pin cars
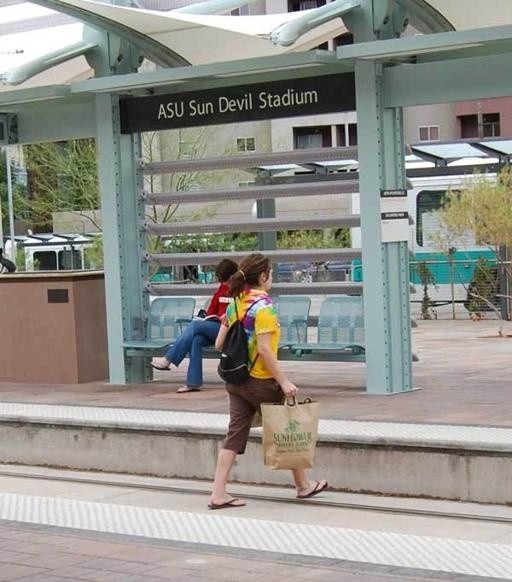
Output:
[277,261,349,281]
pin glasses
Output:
[218,277,227,281]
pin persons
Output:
[149,257,241,394]
[204,252,330,509]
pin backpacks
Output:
[218,296,263,387]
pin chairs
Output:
[120,296,366,363]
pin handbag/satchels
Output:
[260,395,321,470]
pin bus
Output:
[1,231,220,284]
[349,173,500,312]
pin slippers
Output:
[208,496,247,510]
[150,361,171,371]
[297,478,328,499]
[176,386,200,393]
